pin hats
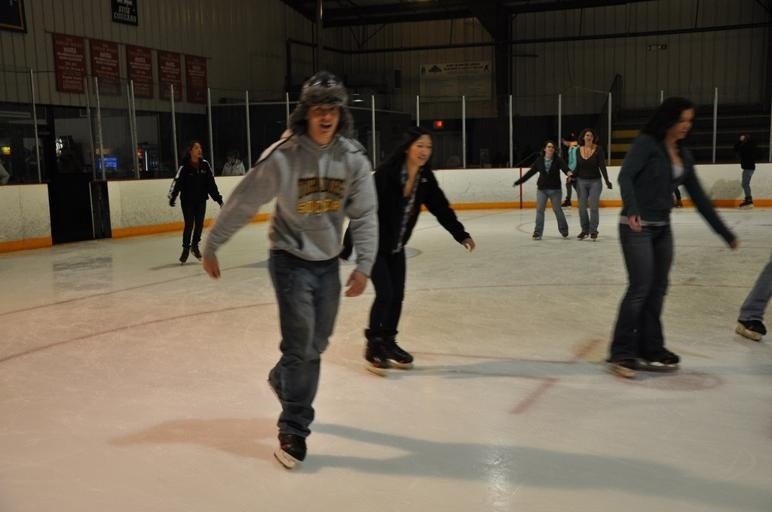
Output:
[289,72,354,139]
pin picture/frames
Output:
[0,0,27,33]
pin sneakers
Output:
[561,200,571,207]
[387,336,413,363]
[278,432,306,461]
[640,347,679,365]
[578,232,588,238]
[609,355,640,371]
[365,335,392,369]
[191,243,202,258]
[737,320,767,335]
[591,234,597,238]
[180,249,189,262]
[740,196,752,207]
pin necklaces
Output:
[582,144,593,156]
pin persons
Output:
[672,188,684,206]
[221,146,246,175]
[571,127,612,238]
[735,132,755,208]
[603,96,741,371]
[26,144,44,170]
[735,258,770,335]
[202,69,382,461]
[338,127,475,370]
[511,140,574,237]
[166,139,225,263]
[560,135,580,207]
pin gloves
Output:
[568,175,574,184]
[607,183,612,189]
